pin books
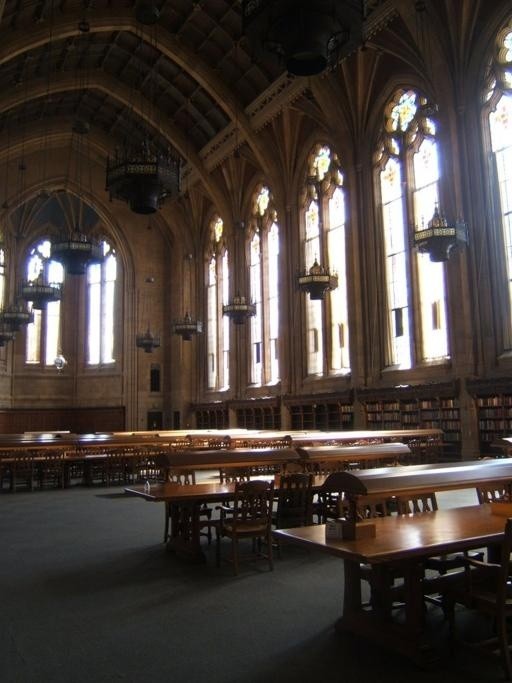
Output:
[342,396,511,442]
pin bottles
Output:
[144,481,151,490]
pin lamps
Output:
[139,319,165,355]
[106,1,182,216]
[218,286,262,326]
[291,253,344,306]
[0,41,60,345]
[169,306,205,346]
[406,196,469,269]
[49,1,109,277]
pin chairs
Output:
[135,491,338,579]
[0,433,512,491]
[339,491,511,683]
